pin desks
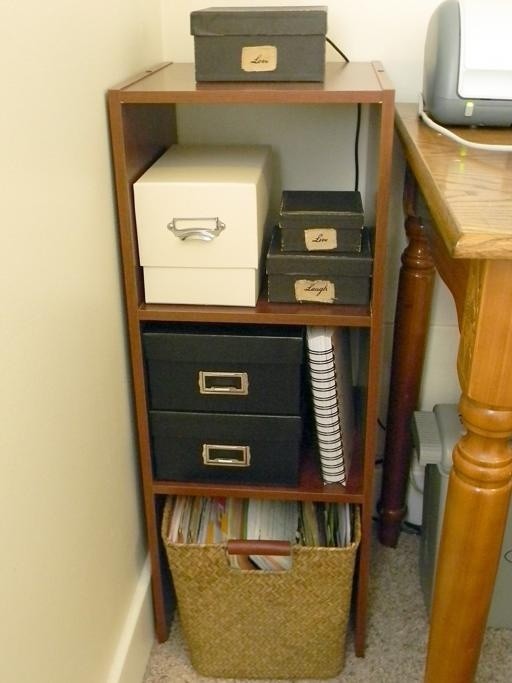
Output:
[376,98,512,683]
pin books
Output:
[308,326,355,487]
[166,494,352,573]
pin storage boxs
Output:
[192,6,327,82]
[147,411,305,491]
[281,190,365,253]
[133,142,271,309]
[264,225,373,308]
[141,330,306,417]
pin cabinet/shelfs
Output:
[105,60,395,504]
